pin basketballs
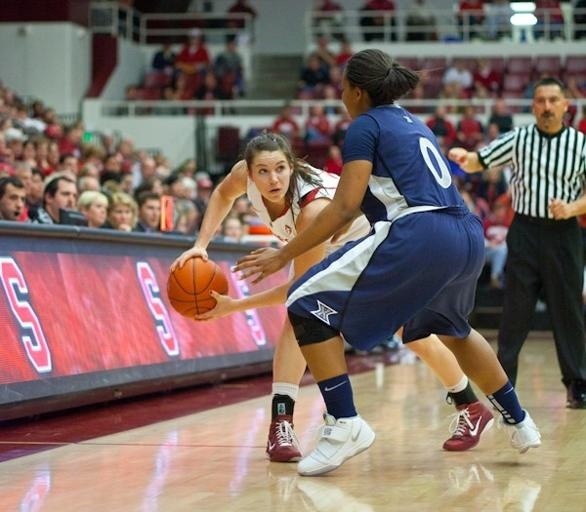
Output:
[167,257,229,318]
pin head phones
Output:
[0,179,20,217]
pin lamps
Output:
[511,14,537,27]
[511,2,536,12]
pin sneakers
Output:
[266,415,302,461]
[298,412,376,476]
[566,384,586,409]
[498,409,541,453]
[443,401,494,451]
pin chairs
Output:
[126,50,586,99]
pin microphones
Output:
[28,208,44,223]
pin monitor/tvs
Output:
[59,208,88,226]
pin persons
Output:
[0,0,586,292]
[447,74,586,409]
[230,47,543,477]
[170,132,495,463]
